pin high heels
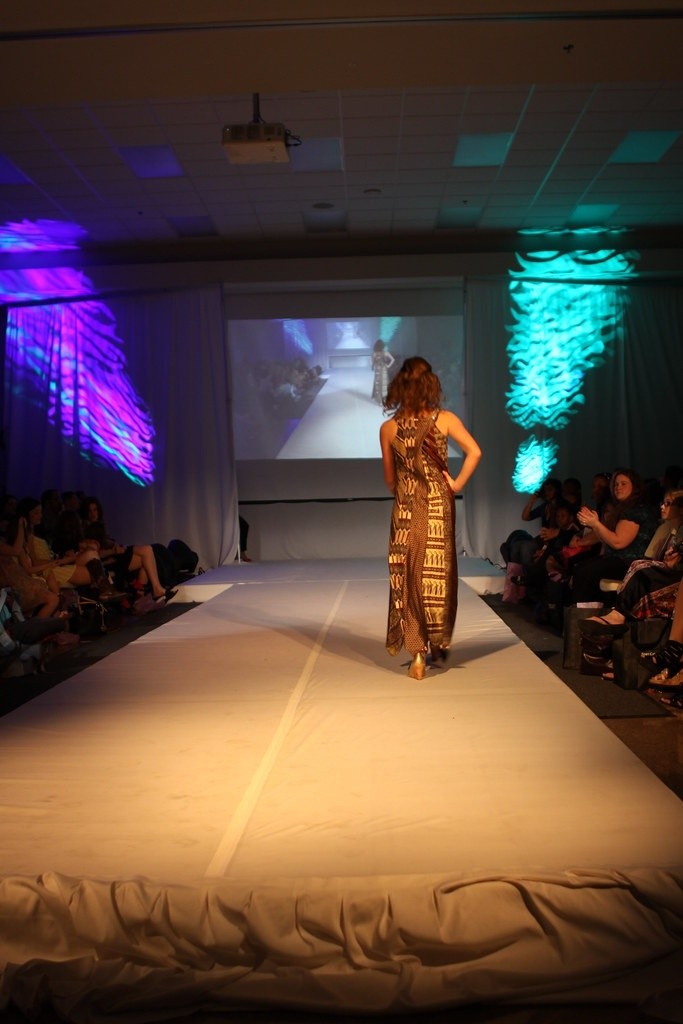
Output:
[408,652,426,680]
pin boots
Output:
[85,558,128,601]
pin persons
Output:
[239,515,251,562]
[0,489,179,655]
[370,339,396,406]
[500,467,683,696]
[380,356,482,681]
[239,357,322,401]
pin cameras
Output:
[602,472,613,480]
[535,491,546,498]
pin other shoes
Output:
[241,557,250,563]
[153,589,178,605]
[502,562,682,717]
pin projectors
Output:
[221,123,291,164]
